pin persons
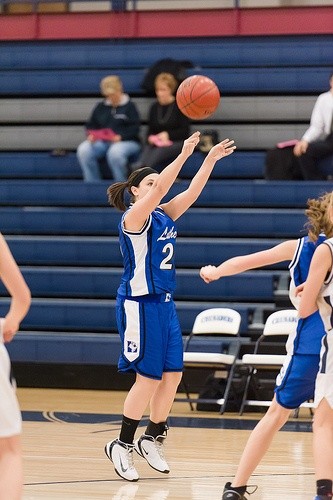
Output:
[76,75,143,184]
[0,230,32,500]
[294,71,333,181]
[294,192,333,500]
[131,72,191,174]
[200,230,327,498]
[105,130,237,481]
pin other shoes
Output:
[221,482,258,500]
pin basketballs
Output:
[175,74,221,121]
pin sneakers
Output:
[133,433,170,474]
[104,437,140,482]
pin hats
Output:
[100,76,121,95]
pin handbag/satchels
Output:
[264,150,293,179]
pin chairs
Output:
[239,309,313,419]
[182,308,243,415]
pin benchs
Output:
[0,35,333,392]
